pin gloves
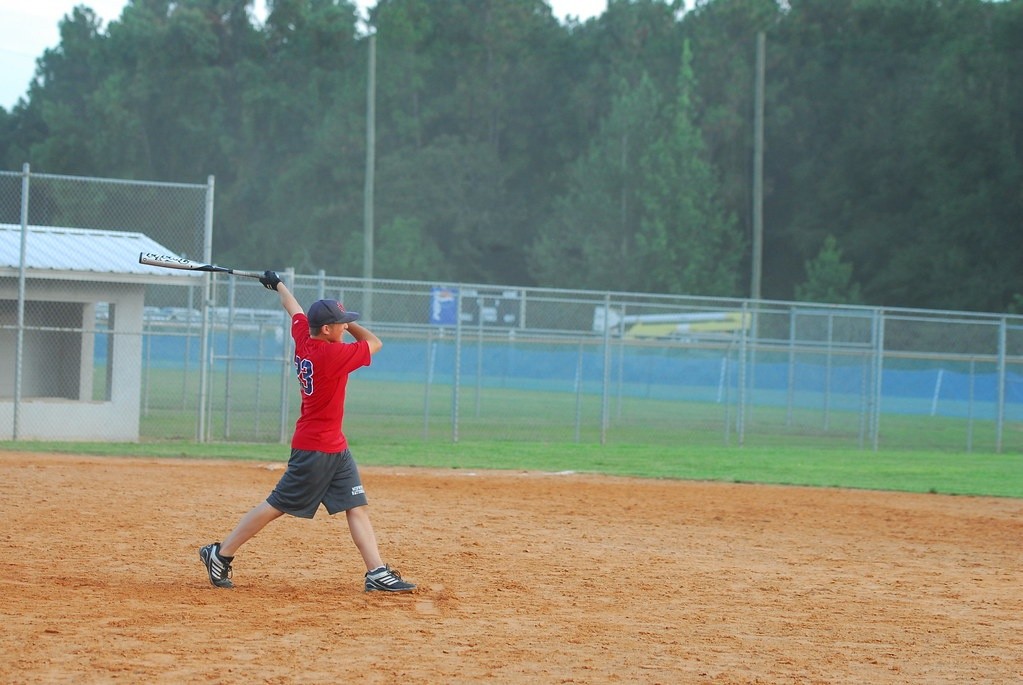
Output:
[259,269,282,292]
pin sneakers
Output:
[199,543,235,588]
[364,563,415,593]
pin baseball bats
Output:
[138,251,280,281]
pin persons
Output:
[200,268,418,591]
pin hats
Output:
[307,299,359,327]
